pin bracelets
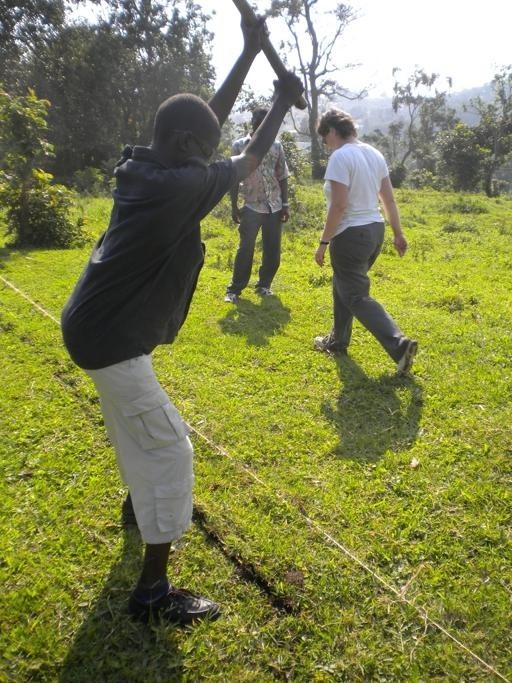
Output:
[283,202,288,209]
[318,240,330,244]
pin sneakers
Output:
[254,287,274,297]
[224,290,238,303]
[128,586,220,628]
[122,499,135,515]
[314,335,347,353]
[395,339,419,377]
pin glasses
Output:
[173,129,215,162]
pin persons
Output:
[312,106,420,378]
[59,10,311,627]
[223,106,292,305]
